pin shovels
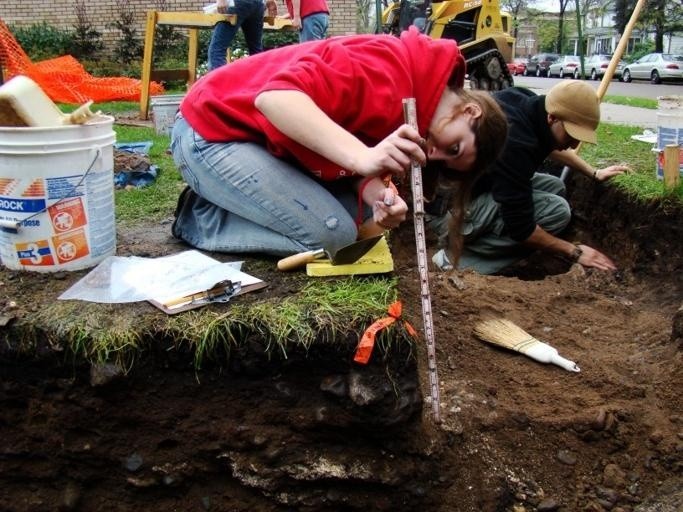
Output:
[277,235,382,272]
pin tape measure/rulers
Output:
[402,98,440,423]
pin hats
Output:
[544,79,600,146]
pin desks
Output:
[139,6,295,119]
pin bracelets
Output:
[569,245,583,262]
[590,169,600,181]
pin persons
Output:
[167,24,513,270]
[206,0,277,71]
[270,1,332,42]
[421,78,636,279]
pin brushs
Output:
[473,316,578,374]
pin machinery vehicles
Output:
[373,1,516,90]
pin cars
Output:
[506,57,529,76]
[546,54,588,79]
[572,53,629,81]
[522,51,559,77]
[621,52,682,84]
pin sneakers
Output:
[430,248,453,271]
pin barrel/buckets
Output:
[149,94,188,136]
[0,113,117,274]
[656,97,683,180]
